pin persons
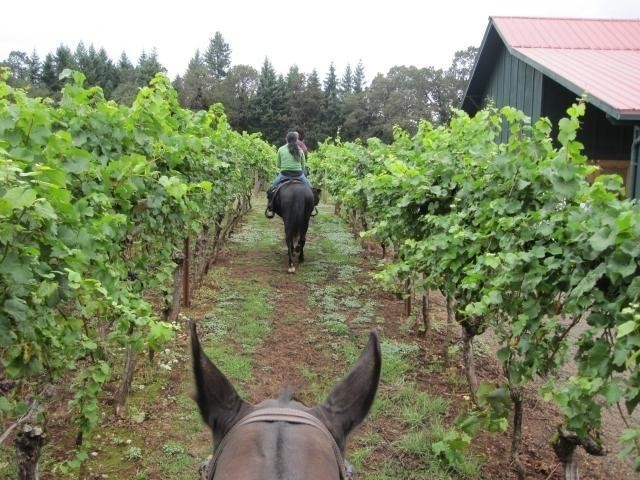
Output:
[293,131,308,156]
[266,132,315,215]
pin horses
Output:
[272,166,315,274]
[187,317,381,480]
